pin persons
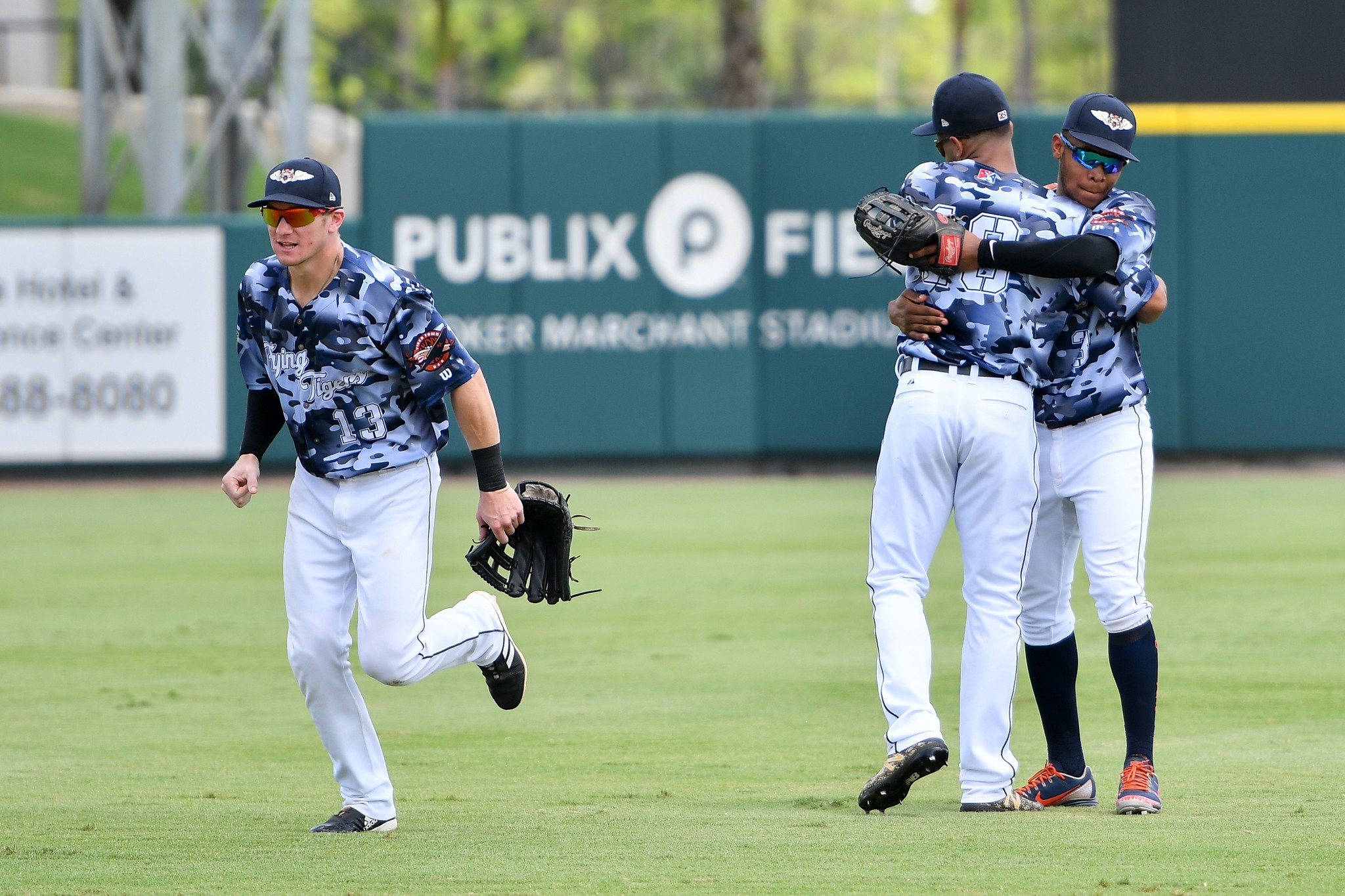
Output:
[854,73,1166,814]
[220,157,527,834]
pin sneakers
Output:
[858,737,949,813]
[960,789,1045,813]
[1014,760,1098,807]
[1116,760,1161,814]
[309,807,398,834]
[467,591,527,709]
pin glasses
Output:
[1060,132,1129,174]
[259,206,344,228]
[933,135,970,157]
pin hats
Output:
[247,157,341,208]
[911,72,1010,136]
[1061,93,1140,163]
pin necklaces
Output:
[288,249,340,309]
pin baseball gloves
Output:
[854,191,966,276]
[467,481,573,605]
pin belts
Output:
[1039,397,1142,430]
[900,357,1028,383]
